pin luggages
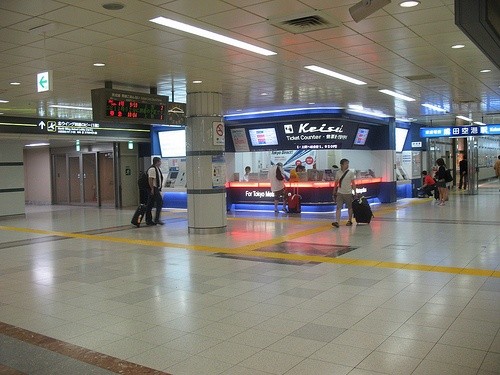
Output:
[352,195,374,224]
[288,181,301,214]
[131,195,153,227]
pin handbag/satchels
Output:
[276,166,284,180]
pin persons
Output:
[432,158,447,206]
[241,166,251,182]
[145,157,166,225]
[420,171,436,198]
[288,165,306,182]
[331,165,339,170]
[493,155,500,192]
[458,154,468,192]
[331,159,357,228]
[268,162,288,216]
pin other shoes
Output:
[283,208,287,213]
[441,198,448,201]
[146,222,157,226]
[275,210,279,213]
[346,221,352,226]
[332,222,339,228]
[154,221,164,225]
[437,201,446,206]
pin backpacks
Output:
[138,166,159,196]
[441,164,453,182]
